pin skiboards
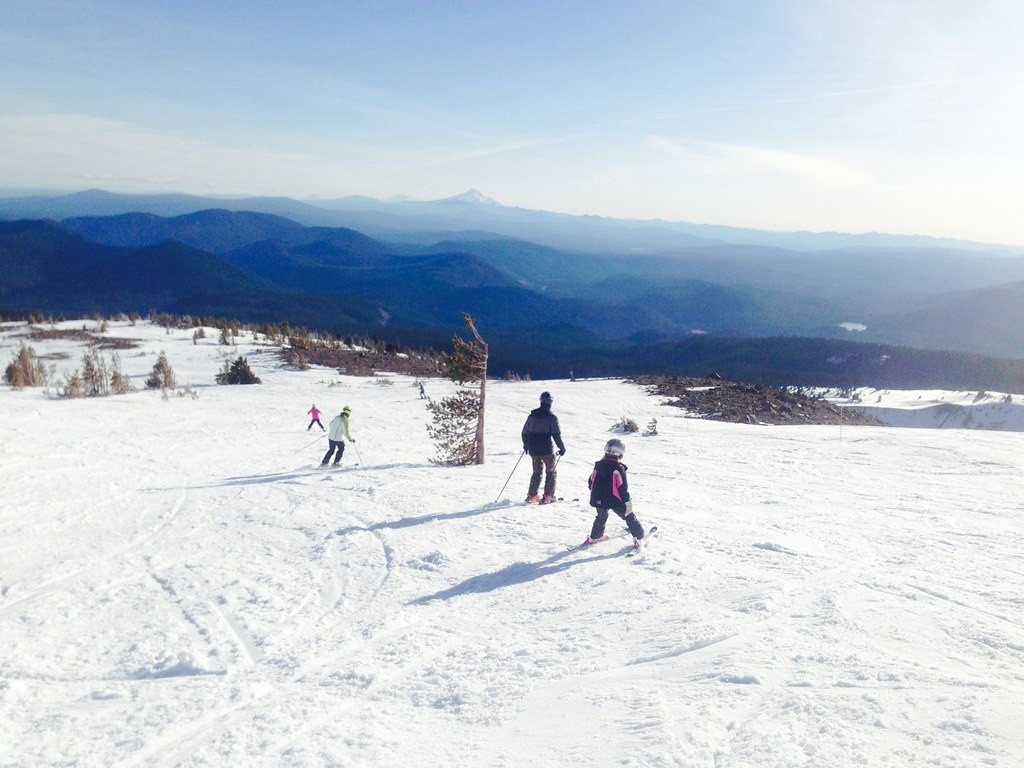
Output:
[415,396,430,401]
[305,427,327,435]
[523,492,579,507]
[566,523,659,558]
[315,462,360,471]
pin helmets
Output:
[540,391,552,404]
[604,439,625,456]
[343,406,351,416]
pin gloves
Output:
[559,449,565,456]
[524,445,529,454]
[352,439,355,442]
[625,501,632,516]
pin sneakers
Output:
[527,495,540,503]
[589,535,606,542]
[542,494,556,503]
[634,534,647,547]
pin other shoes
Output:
[334,462,342,466]
[322,463,330,467]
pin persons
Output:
[520,391,564,501]
[418,382,426,399]
[307,404,327,432]
[586,438,649,547]
[320,403,355,468]
